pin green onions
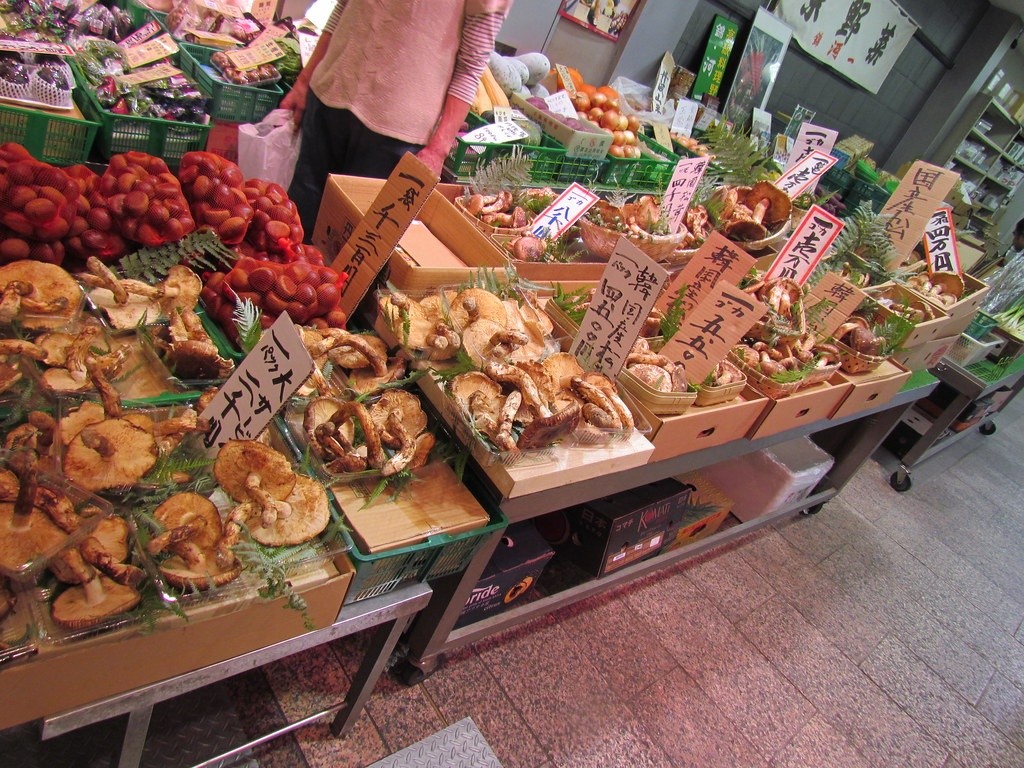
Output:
[993,297,1024,335]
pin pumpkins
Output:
[473,51,620,146]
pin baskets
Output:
[444,110,680,188]
[272,413,509,605]
[944,335,1002,367]
[855,159,879,184]
[0,301,246,427]
[0,0,293,166]
[455,192,894,413]
[964,312,998,339]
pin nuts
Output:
[0,140,345,343]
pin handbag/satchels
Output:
[236,108,302,193]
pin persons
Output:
[274,0,512,334]
[1013,219,1024,252]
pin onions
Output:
[670,132,716,158]
[525,90,641,157]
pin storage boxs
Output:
[0,0,1024,728]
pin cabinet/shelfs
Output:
[929,91,1024,211]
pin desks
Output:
[400,370,940,685]
[890,356,1024,493]
[39,578,433,768]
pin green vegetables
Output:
[273,40,302,75]
[69,38,194,122]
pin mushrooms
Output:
[211,51,279,84]
[293,289,635,476]
[468,181,956,392]
[0,256,433,627]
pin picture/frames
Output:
[721,5,794,134]
